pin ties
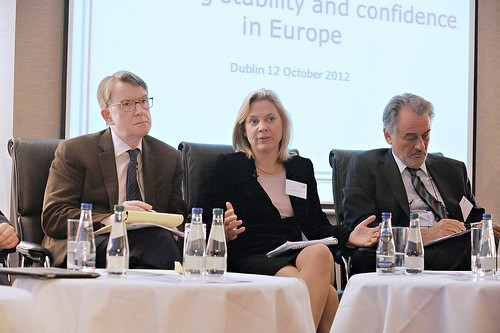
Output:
[405,166,449,219]
[126,149,143,202]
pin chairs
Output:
[7,137,64,267]
[330,149,444,291]
[178,142,350,285]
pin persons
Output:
[341,93,500,280]
[187,88,383,333]
[0,212,21,287]
[42,70,193,270]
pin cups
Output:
[392,226,408,276]
[470,222,482,280]
[184,223,206,259]
[66,218,84,271]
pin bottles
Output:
[376,213,396,276]
[404,213,424,277]
[105,205,130,279]
[183,208,206,280]
[476,213,496,281]
[72,203,96,273]
[204,208,228,282]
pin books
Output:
[422,224,497,246]
[93,210,185,237]
[266,236,338,258]
[0,266,101,279]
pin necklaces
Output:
[256,166,273,175]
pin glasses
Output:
[104,98,153,112]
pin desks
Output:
[330,271,499,333]
[12,268,315,333]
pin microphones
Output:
[252,174,256,178]
[136,162,138,170]
[435,199,442,204]
